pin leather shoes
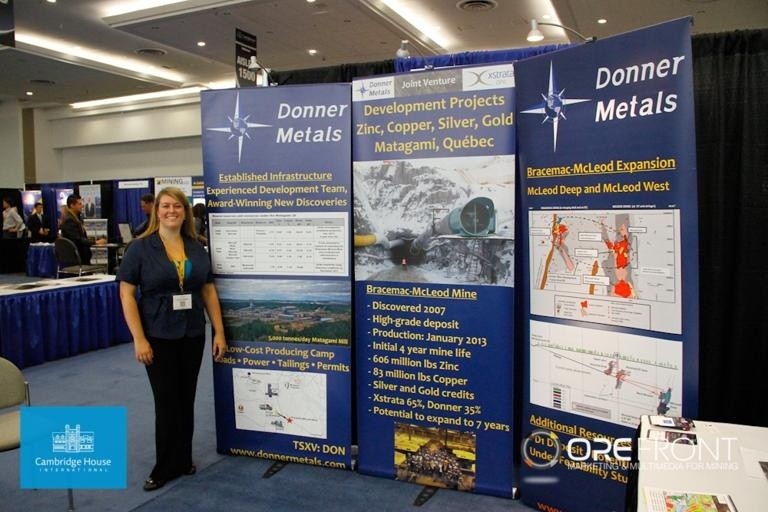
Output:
[143,464,197,491]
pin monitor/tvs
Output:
[115,221,135,245]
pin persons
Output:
[85,197,95,217]
[0,197,51,244]
[116,187,229,490]
[56,195,107,265]
[133,193,209,250]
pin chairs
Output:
[53,238,106,278]
[0,358,34,451]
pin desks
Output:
[636,414,768,511]
[0,273,134,364]
